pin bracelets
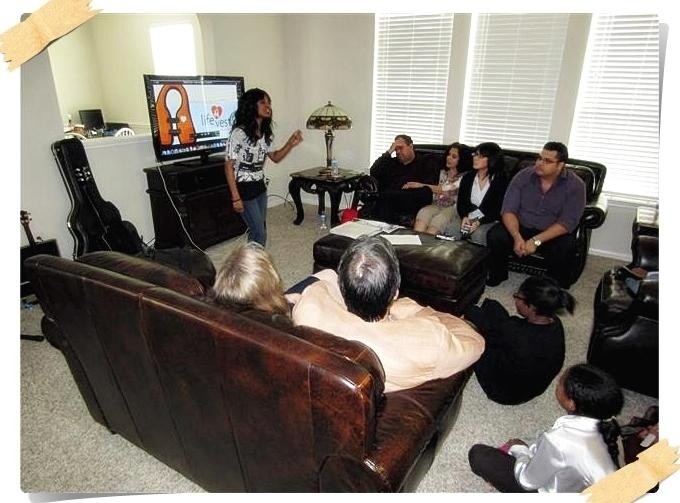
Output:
[232,197,241,203]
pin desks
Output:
[313,227,492,318]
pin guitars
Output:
[20,211,40,254]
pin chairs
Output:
[114,127,136,138]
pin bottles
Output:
[319,210,327,229]
[331,160,338,175]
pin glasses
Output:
[533,154,558,162]
[513,292,533,304]
[472,151,490,159]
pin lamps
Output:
[306,100,353,174]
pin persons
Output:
[445,142,508,245]
[469,363,625,493]
[485,142,586,288]
[292,235,485,392]
[148,246,216,293]
[370,136,433,229]
[224,87,303,249]
[465,275,575,405]
[403,143,473,242]
[209,244,336,322]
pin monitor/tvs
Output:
[79,110,105,129]
[143,75,245,167]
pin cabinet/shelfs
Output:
[143,155,249,250]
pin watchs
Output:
[533,236,542,248]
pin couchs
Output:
[586,235,659,400]
[358,145,607,285]
[23,249,473,493]
